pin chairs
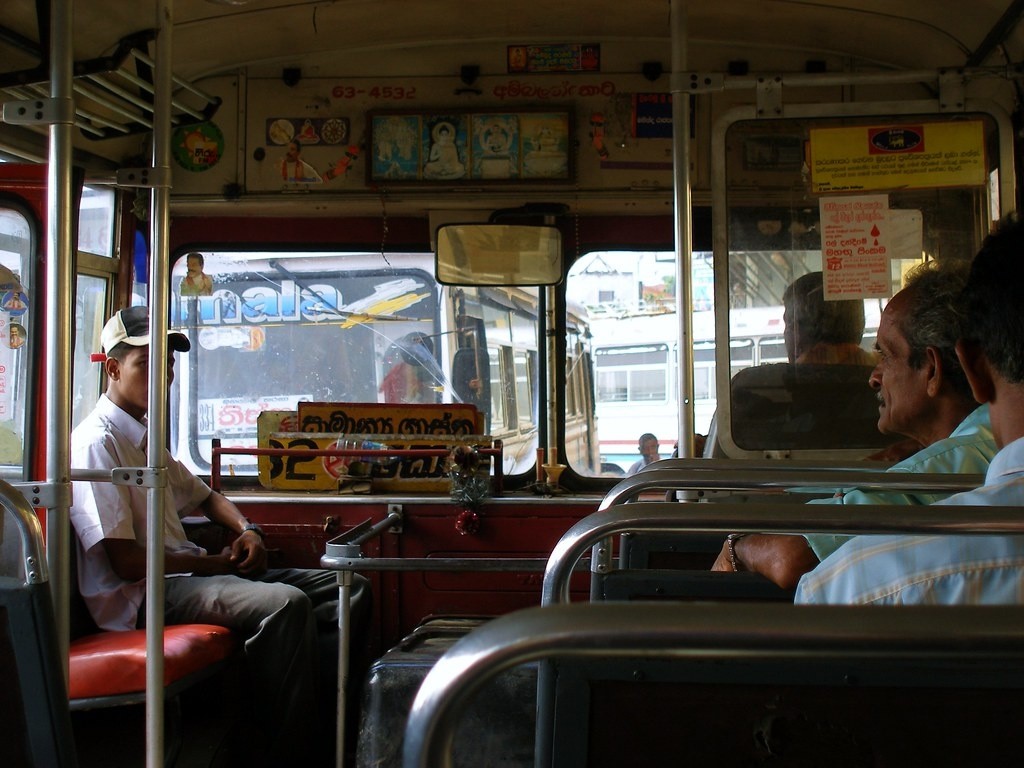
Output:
[69,516,236,768]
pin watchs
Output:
[728,534,746,572]
[241,523,265,539]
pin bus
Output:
[590,298,889,478]
[170,250,600,477]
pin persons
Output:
[10,326,24,348]
[783,272,882,365]
[181,253,213,295]
[628,434,661,475]
[5,293,26,308]
[68,305,371,768]
[710,259,1001,588]
[794,218,1024,607]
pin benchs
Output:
[401,366,1024,767]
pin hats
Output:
[100,306,194,360]
